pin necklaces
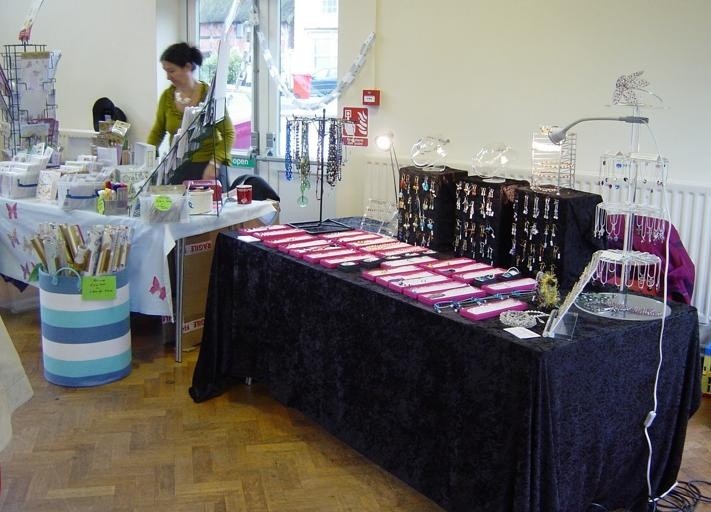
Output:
[599,152,668,201]
[426,293,447,298]
[174,85,199,106]
[591,250,661,290]
[500,310,551,328]
[578,294,662,317]
[367,242,402,251]
[398,279,427,286]
[476,267,519,282]
[442,269,456,273]
[595,202,667,245]
[285,113,345,207]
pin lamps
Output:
[546,115,648,146]
[376,131,400,207]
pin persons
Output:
[146,42,235,194]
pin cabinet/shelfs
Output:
[187,215,702,512]
[0,193,281,363]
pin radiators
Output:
[361,155,709,323]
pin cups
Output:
[237,185,252,204]
